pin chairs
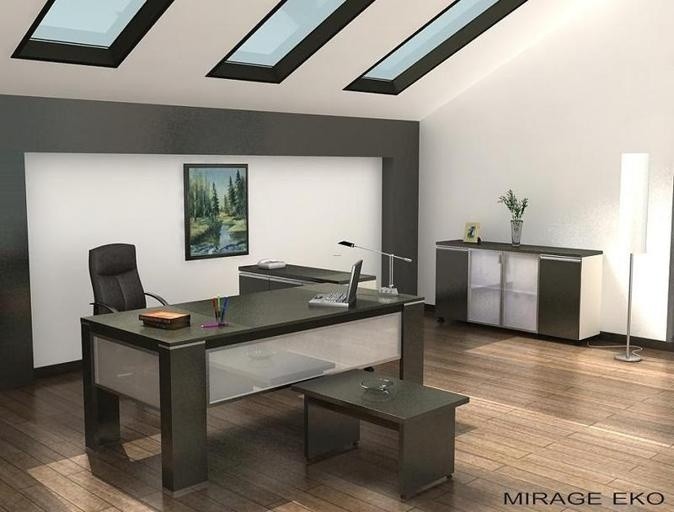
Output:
[87,243,170,313]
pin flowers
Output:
[497,184,528,243]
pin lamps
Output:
[338,240,412,295]
[616,152,646,362]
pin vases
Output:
[509,219,522,247]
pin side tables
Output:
[291,370,470,503]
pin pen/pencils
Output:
[201,324,231,328]
[212,297,217,321]
[221,297,229,323]
[218,296,221,323]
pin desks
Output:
[81,282,427,498]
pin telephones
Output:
[257,258,286,269]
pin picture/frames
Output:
[184,162,249,261]
[464,222,480,243]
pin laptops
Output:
[308,260,362,308]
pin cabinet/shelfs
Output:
[238,262,376,392]
[434,239,604,348]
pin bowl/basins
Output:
[361,390,393,402]
[361,378,394,391]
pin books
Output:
[138,309,191,330]
